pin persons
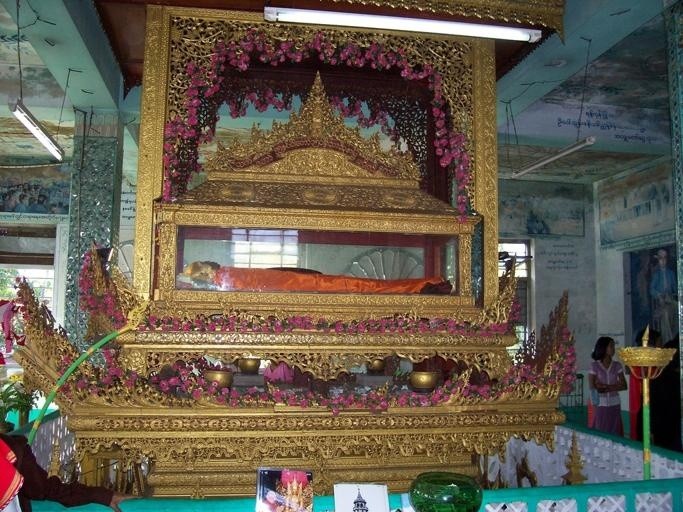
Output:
[183,261,447,295]
[648,250,679,344]
[0,425,143,512]
[635,254,651,330]
[588,335,627,437]
[259,490,279,512]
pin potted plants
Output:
[0,380,19,433]
[10,380,43,428]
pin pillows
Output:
[176,272,218,291]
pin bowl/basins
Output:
[408,371,439,391]
[204,370,233,388]
[238,358,261,374]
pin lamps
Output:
[500,37,596,182]
[7,0,80,162]
[263,0,543,45]
